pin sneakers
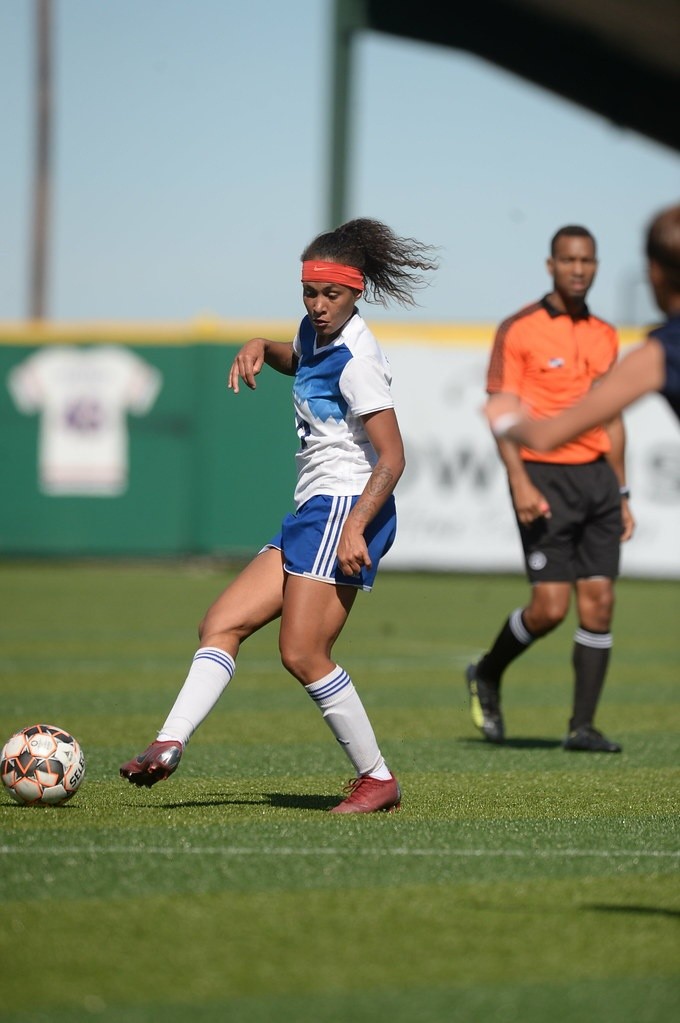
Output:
[119,740,182,788]
[564,723,621,752]
[467,665,504,743]
[329,769,402,813]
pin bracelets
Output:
[620,487,629,497]
[493,412,517,434]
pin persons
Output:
[465,226,635,752]
[121,219,440,812]
[483,209,680,453]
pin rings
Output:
[542,504,549,512]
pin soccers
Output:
[0,723,86,808]
